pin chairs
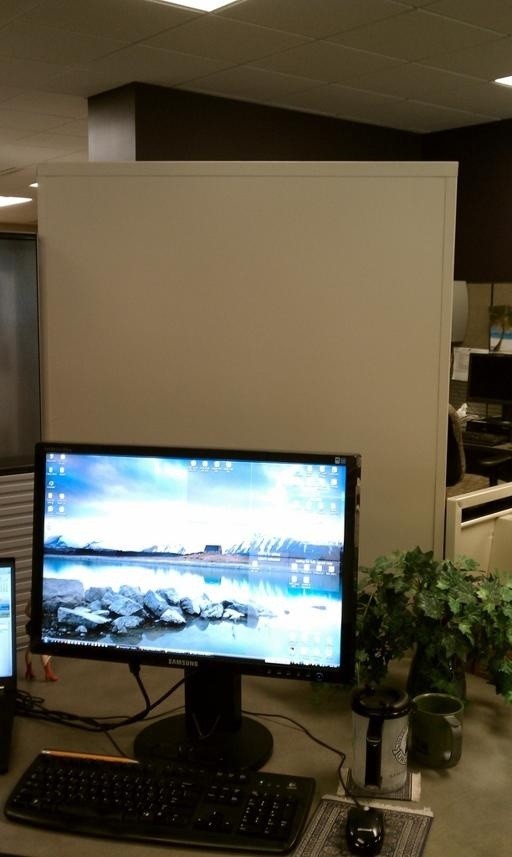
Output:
[446,403,512,497]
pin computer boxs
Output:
[466,420,512,436]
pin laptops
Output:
[0,556,18,776]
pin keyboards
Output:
[461,431,507,446]
[3,751,317,854]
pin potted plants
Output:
[358,544,512,706]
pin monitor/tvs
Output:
[466,352,512,424]
[30,442,361,771]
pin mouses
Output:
[344,807,384,857]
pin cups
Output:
[351,685,466,795]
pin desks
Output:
[0,647,511,856]
[460,414,512,455]
